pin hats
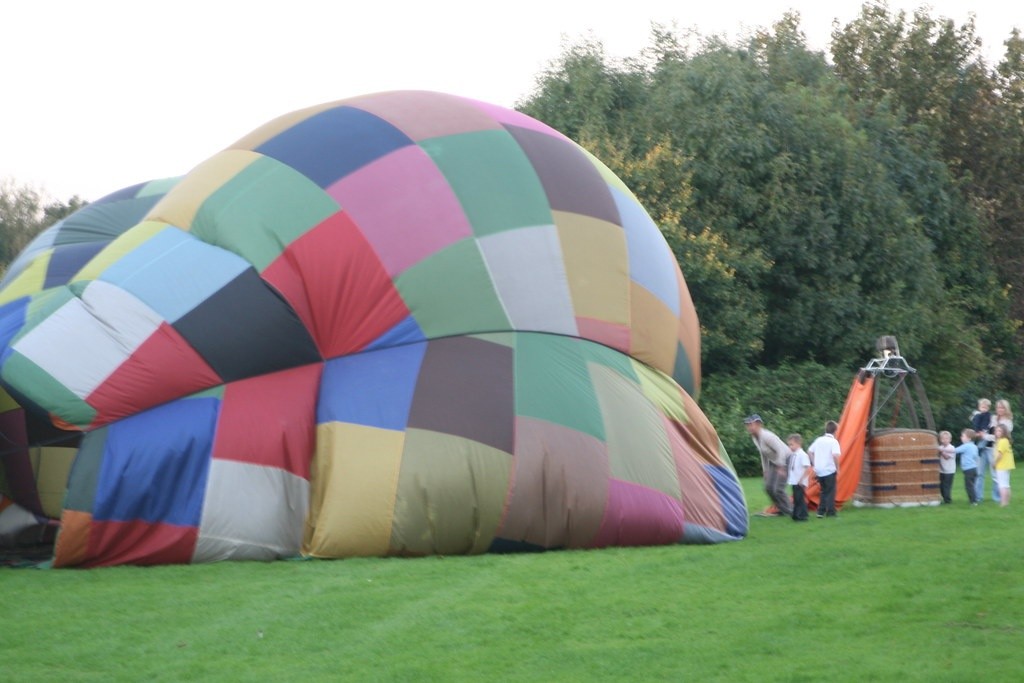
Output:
[744,414,762,424]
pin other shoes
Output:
[817,513,823,518]
[972,502,977,505]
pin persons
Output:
[807,420,841,518]
[742,414,793,517]
[938,430,956,504]
[992,423,1016,506]
[968,398,1013,502]
[786,433,811,521]
[938,428,978,505]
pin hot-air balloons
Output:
[0,92,942,570]
